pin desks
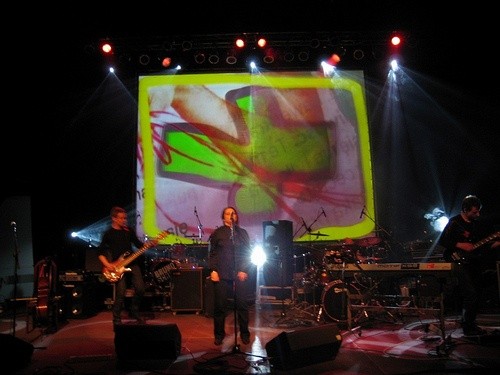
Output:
[11,298,45,342]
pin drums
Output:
[321,279,362,322]
[324,250,353,280]
[359,237,387,260]
[148,257,181,294]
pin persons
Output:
[97,207,159,331]
[207,207,251,345]
[440,195,500,337]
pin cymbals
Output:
[136,235,157,239]
[294,245,320,258]
[185,235,203,238]
[307,233,330,236]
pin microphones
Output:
[11,220,18,233]
[321,207,327,217]
[359,208,364,218]
[301,216,311,232]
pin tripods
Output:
[273,216,421,336]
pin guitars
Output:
[442,230,500,276]
[101,229,171,282]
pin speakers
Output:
[84,248,113,272]
[169,270,203,316]
[60,281,97,319]
[264,324,342,370]
[114,322,181,371]
[0,333,34,375]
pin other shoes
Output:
[241,336,249,344]
[214,337,222,345]
[464,326,488,335]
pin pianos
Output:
[323,260,453,331]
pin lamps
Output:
[139,33,365,65]
